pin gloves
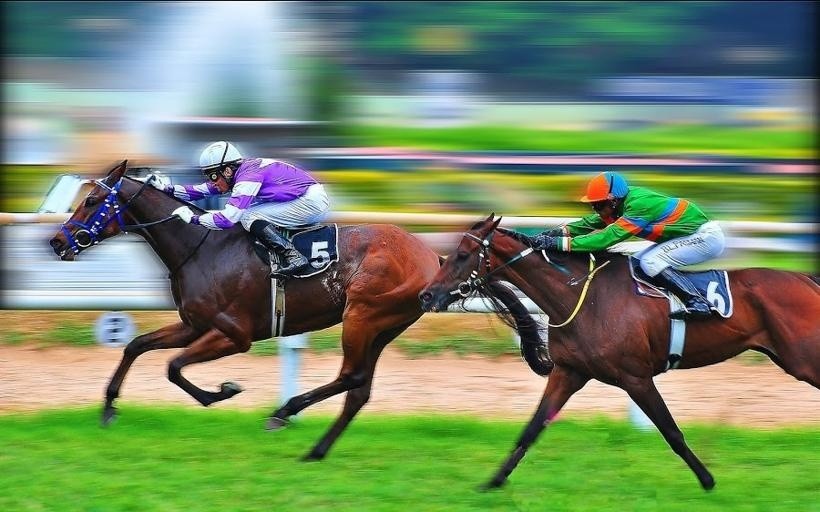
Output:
[528,228,562,250]
[147,171,171,191]
[172,206,194,224]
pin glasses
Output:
[209,162,227,182]
[592,201,610,211]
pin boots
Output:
[256,223,309,279]
[655,267,712,320]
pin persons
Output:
[537,171,725,318]
[150,141,331,273]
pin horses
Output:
[417,212,819,492]
[50,158,555,463]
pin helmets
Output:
[580,171,629,203]
[199,140,243,170]
